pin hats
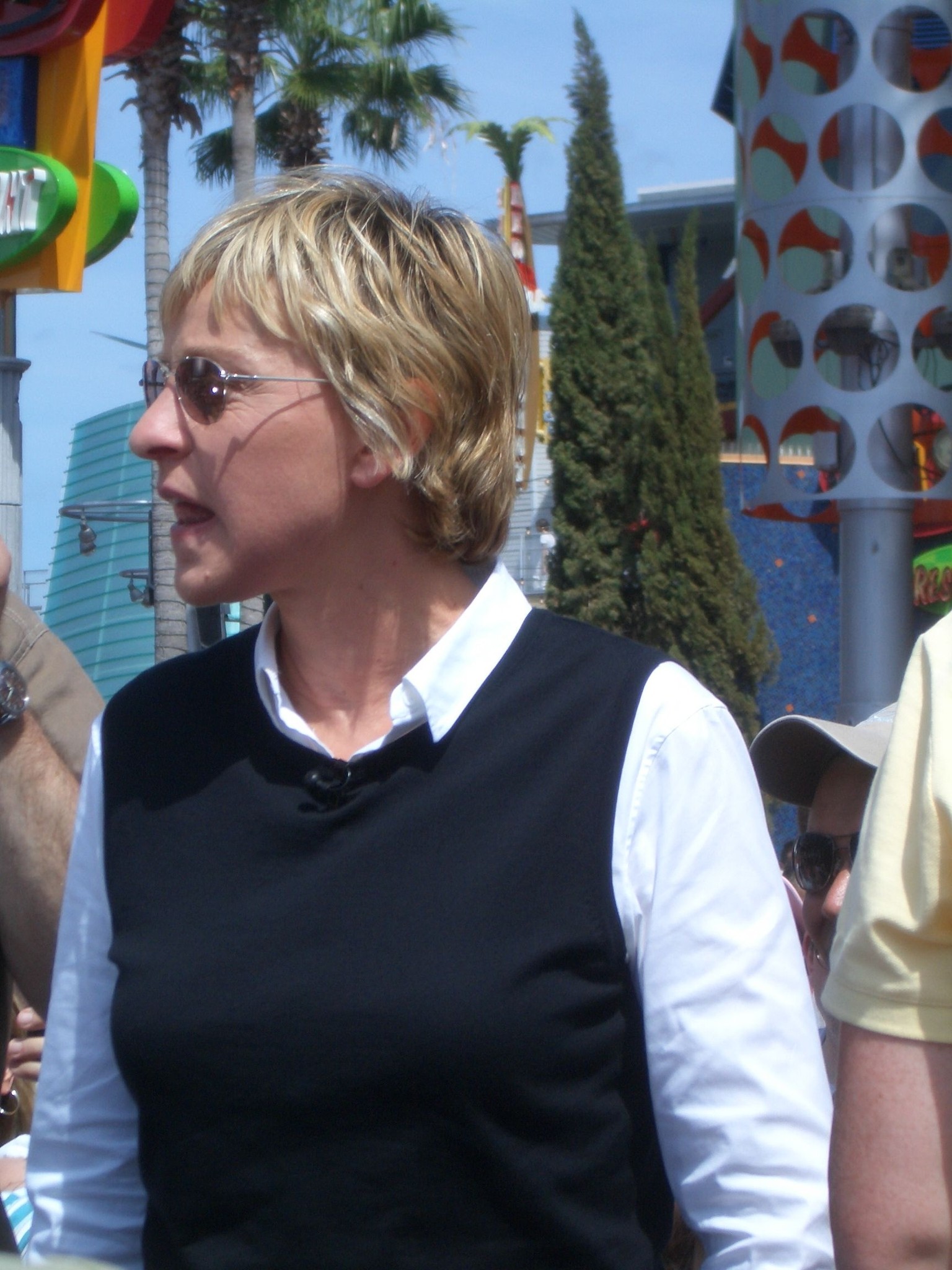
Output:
[750,698,901,807]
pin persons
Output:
[818,594,952,1270]
[0,163,844,1270]
[745,696,899,1099]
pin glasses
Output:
[139,355,333,424]
[790,830,861,893]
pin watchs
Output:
[0,657,30,724]
